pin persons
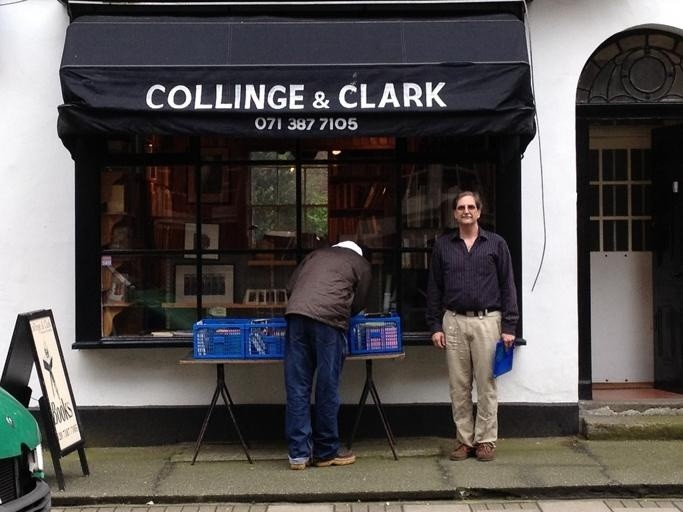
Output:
[282,239,376,470]
[422,191,522,463]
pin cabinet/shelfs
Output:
[396,135,498,332]
[101,136,175,337]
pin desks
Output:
[246,260,297,302]
[161,301,286,331]
[180,348,405,464]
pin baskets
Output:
[191,317,250,360]
[243,316,288,359]
[348,310,403,357]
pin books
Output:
[330,163,491,270]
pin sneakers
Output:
[289,463,305,470]
[473,441,496,460]
[311,449,355,467]
[448,441,473,462]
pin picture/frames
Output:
[186,145,230,204]
[173,222,236,305]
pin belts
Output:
[445,304,499,317]
